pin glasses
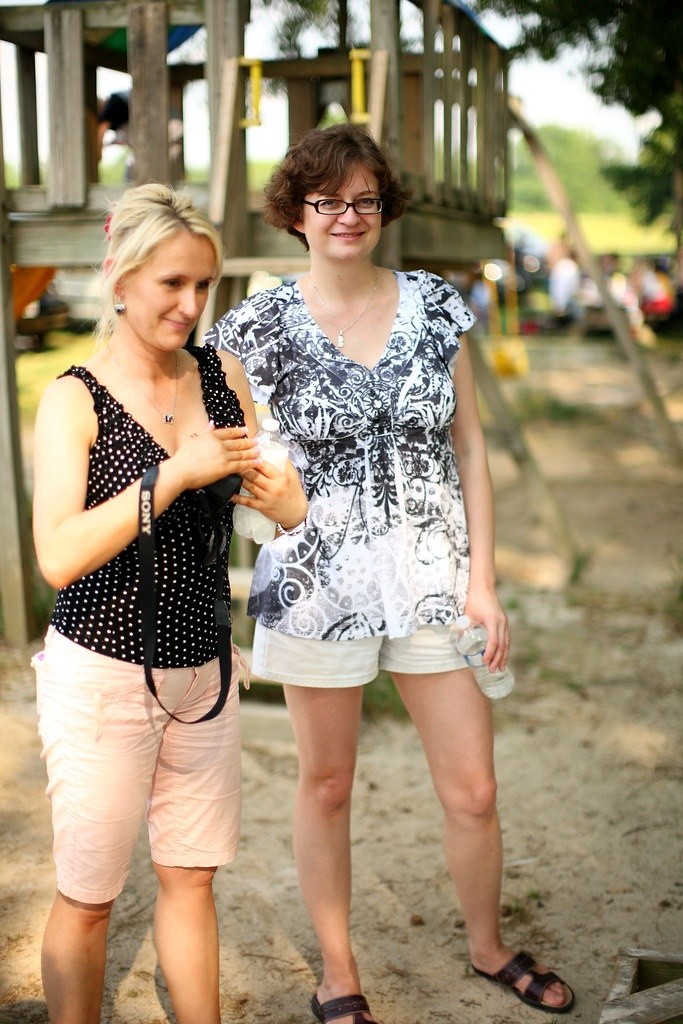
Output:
[301,198,383,215]
[193,484,228,562]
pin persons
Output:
[546,234,683,337]
[206,121,579,1024]
[31,183,309,1024]
[96,93,132,163]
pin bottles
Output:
[454,614,516,700]
[231,418,289,546]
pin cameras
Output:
[205,473,243,512]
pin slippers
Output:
[311,993,378,1024]
[471,951,575,1014]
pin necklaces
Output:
[307,264,379,348]
[100,348,178,425]
